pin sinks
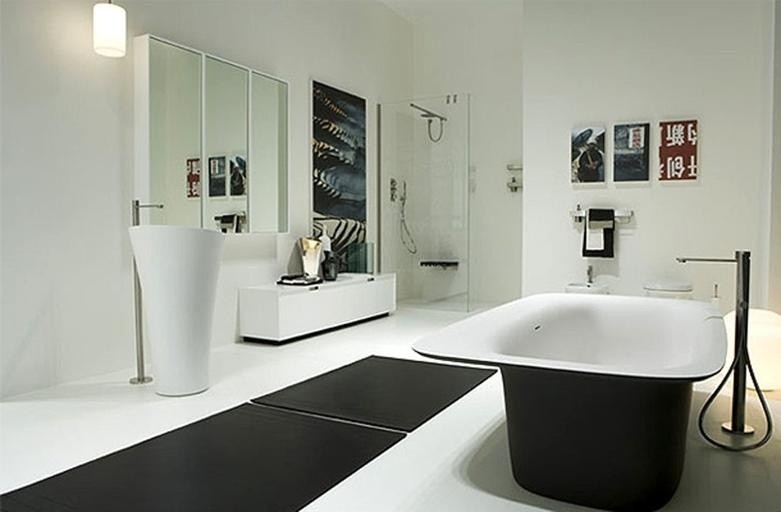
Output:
[130,222,227,395]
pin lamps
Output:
[92,0,127,60]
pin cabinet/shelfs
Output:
[236,272,398,345]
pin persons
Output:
[231,165,244,194]
[578,142,602,181]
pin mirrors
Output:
[133,32,292,238]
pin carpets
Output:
[250,352,499,434]
[1,400,407,509]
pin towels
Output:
[582,207,617,260]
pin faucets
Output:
[675,252,757,435]
[128,199,165,384]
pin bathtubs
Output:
[411,288,725,512]
